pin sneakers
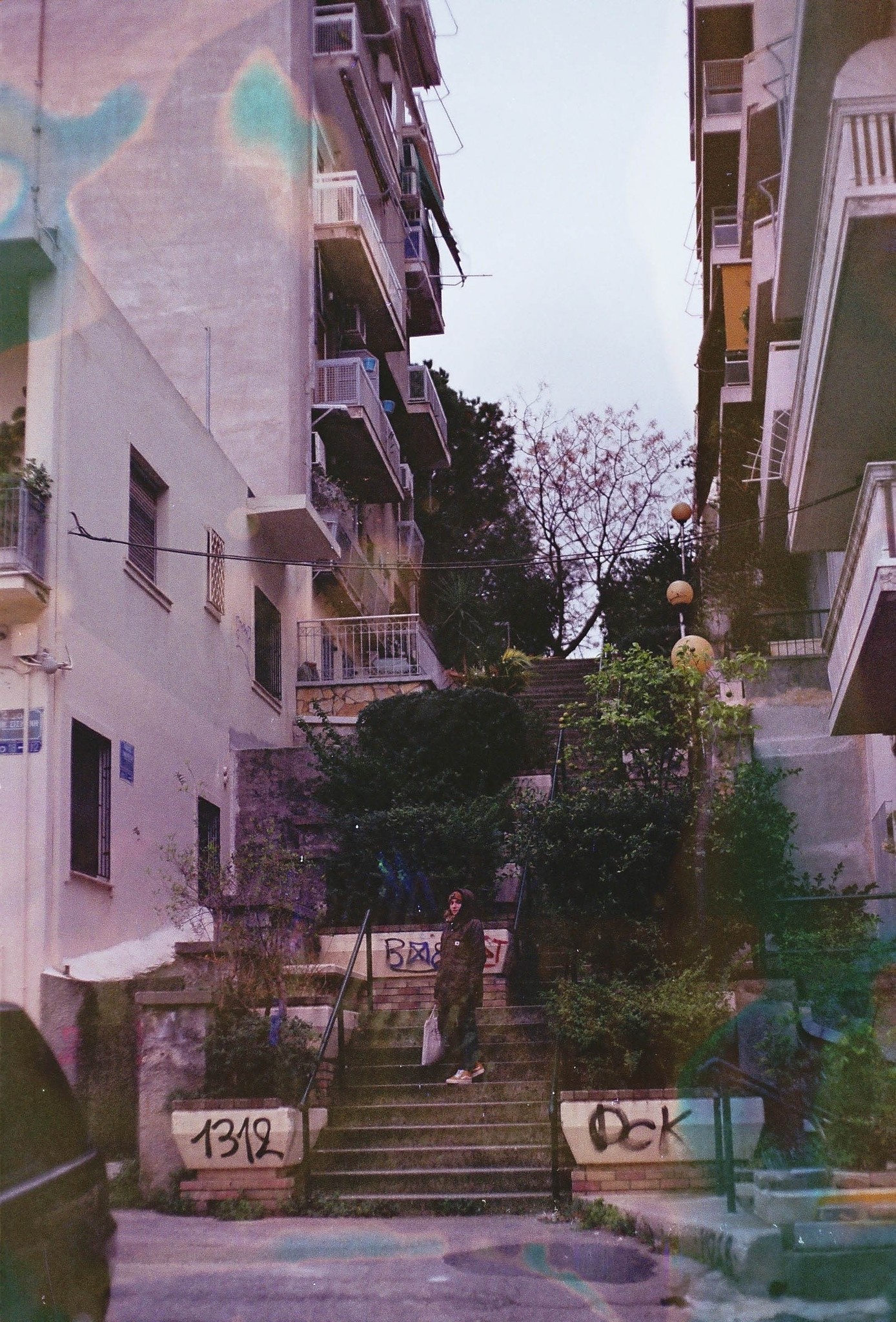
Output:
[447,1070,474,1085]
[472,1061,484,1078]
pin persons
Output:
[433,888,487,1086]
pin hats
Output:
[449,890,462,901]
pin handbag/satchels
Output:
[422,1002,445,1066]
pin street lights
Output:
[667,580,692,639]
[674,635,716,789]
[672,503,692,576]
